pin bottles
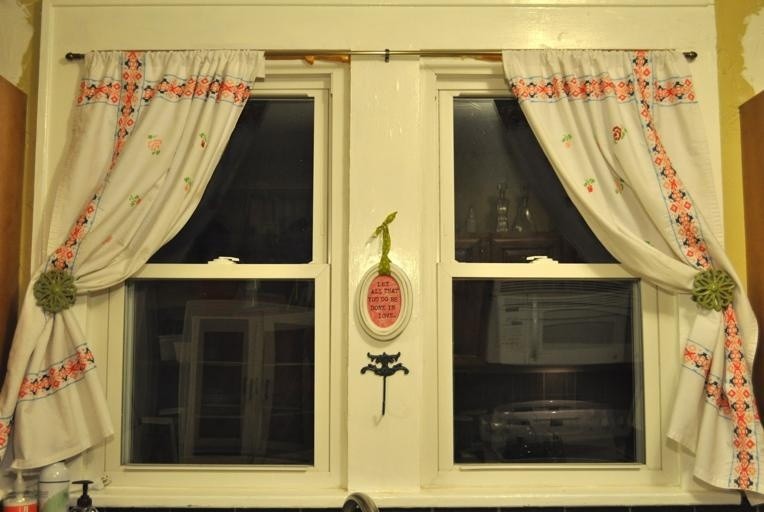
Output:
[38,461,70,512]
[465,182,535,234]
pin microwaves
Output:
[492,297,630,366]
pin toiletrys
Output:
[66,479,100,512]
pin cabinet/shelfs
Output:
[173,297,316,467]
